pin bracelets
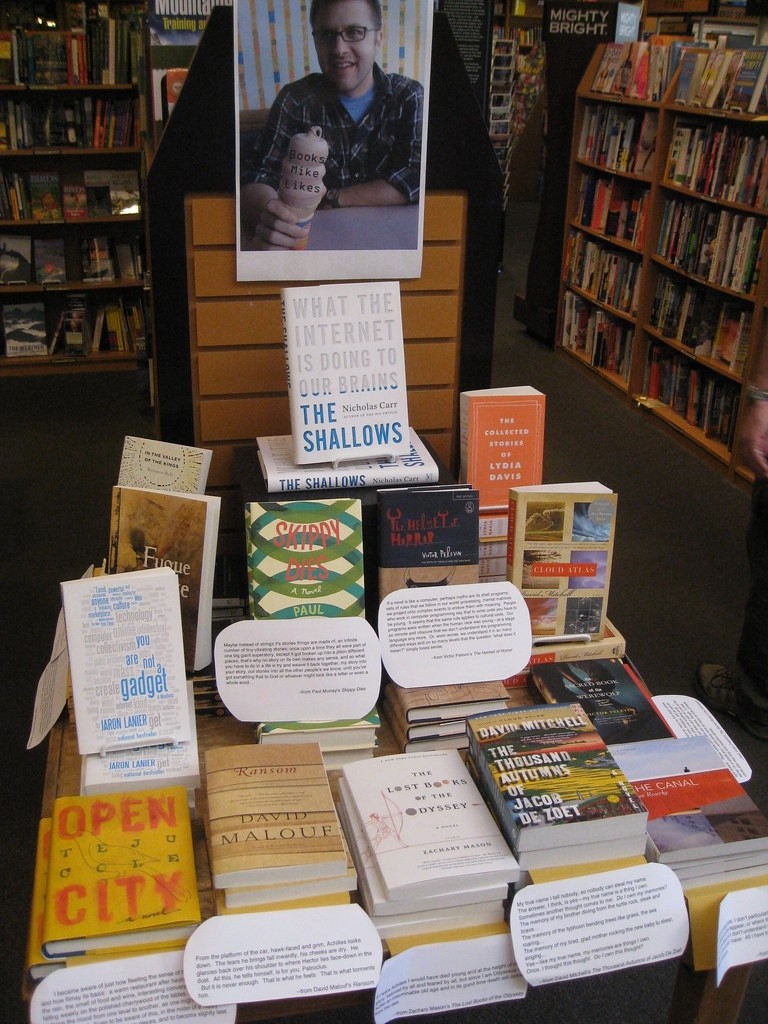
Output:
[744,385,768,401]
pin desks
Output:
[18,677,751,1024]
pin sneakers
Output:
[694,661,768,742]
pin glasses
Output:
[311,27,377,45]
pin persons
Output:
[235,0,423,251]
[696,233,768,742]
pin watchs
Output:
[326,187,340,209]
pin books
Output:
[564,234,753,375]
[503,481,618,641]
[255,424,439,492]
[489,24,542,78]
[246,497,366,620]
[281,281,409,467]
[30,509,768,975]
[378,485,480,612]
[561,292,740,455]
[61,562,190,754]
[107,484,222,672]
[573,173,766,296]
[0,15,189,361]
[577,105,768,212]
[590,35,768,119]
[460,385,546,511]
[118,437,214,496]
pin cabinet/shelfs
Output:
[0,7,158,377]
[488,0,547,213]
[552,34,767,484]
